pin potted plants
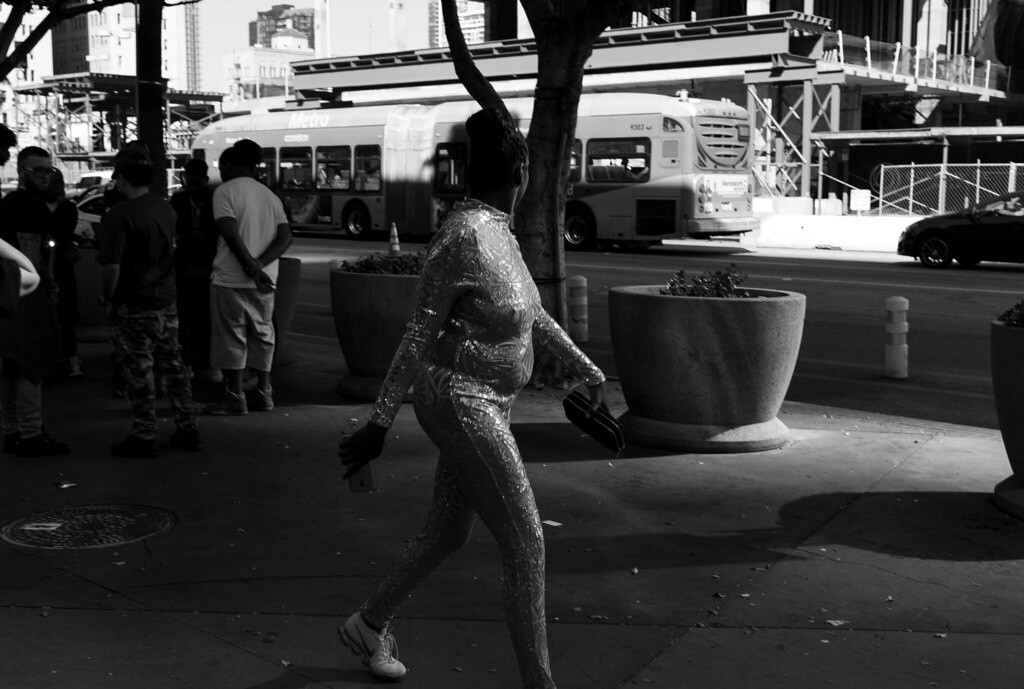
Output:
[328,250,444,380]
[607,261,808,452]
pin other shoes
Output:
[17,432,71,457]
[168,423,202,452]
[3,431,23,453]
[110,435,164,459]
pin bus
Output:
[188,90,756,255]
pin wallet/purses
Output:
[563,391,626,453]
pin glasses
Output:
[18,164,55,178]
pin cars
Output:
[895,194,1023,274]
[70,183,106,250]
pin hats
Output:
[111,144,152,178]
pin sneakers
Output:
[338,609,406,681]
[246,383,274,411]
[202,389,248,416]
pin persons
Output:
[96,146,204,437]
[58,139,70,153]
[0,121,48,356]
[630,160,648,182]
[617,157,632,172]
[332,96,625,687]
[73,138,87,153]
[2,144,80,462]
[993,200,1023,218]
[213,149,251,192]
[42,164,78,301]
[70,141,81,153]
[206,136,295,414]
